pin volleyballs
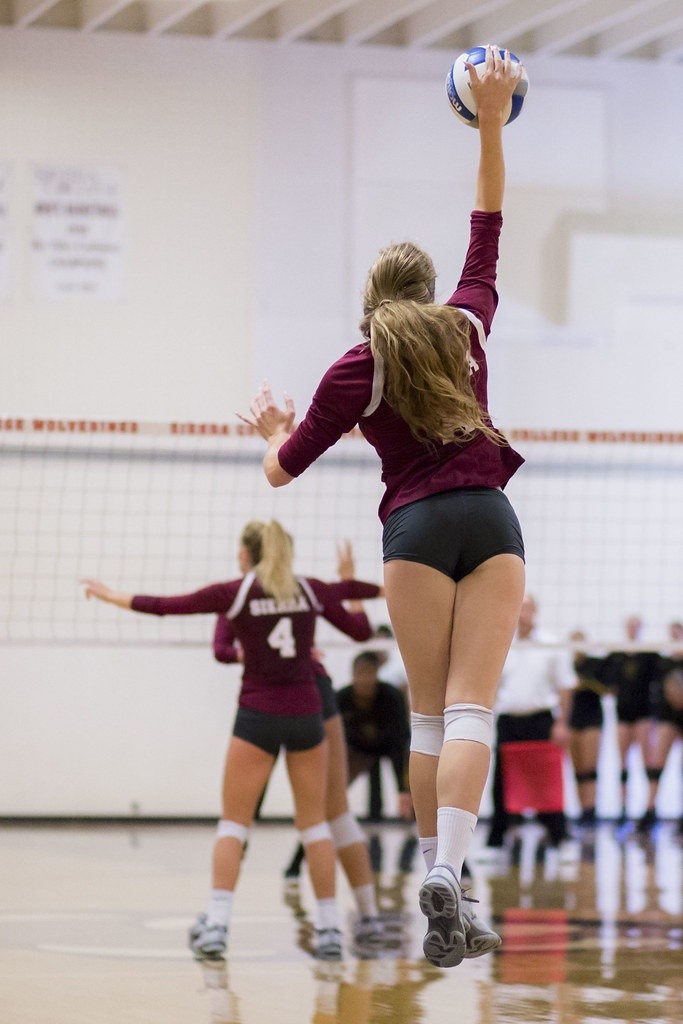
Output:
[445,45,529,129]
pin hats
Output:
[350,647,388,670]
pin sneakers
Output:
[189,913,228,957]
[418,864,466,969]
[308,927,343,961]
[461,898,502,960]
[353,918,386,943]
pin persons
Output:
[78,520,683,961]
[230,44,527,968]
